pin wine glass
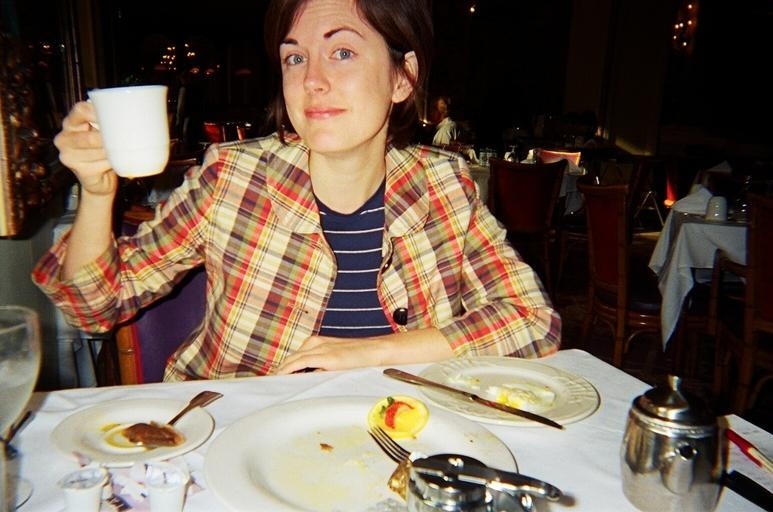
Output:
[0,306,40,510]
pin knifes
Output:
[383,363,570,433]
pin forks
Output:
[367,426,561,500]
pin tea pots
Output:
[621,376,729,512]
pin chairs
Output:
[117,207,208,385]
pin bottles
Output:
[404,451,494,511]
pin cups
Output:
[706,196,728,223]
[57,459,193,512]
[82,83,175,179]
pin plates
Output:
[419,357,605,424]
[202,394,523,512]
[49,396,214,465]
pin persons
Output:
[432,96,461,146]
[29,1,562,383]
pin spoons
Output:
[130,387,223,450]
